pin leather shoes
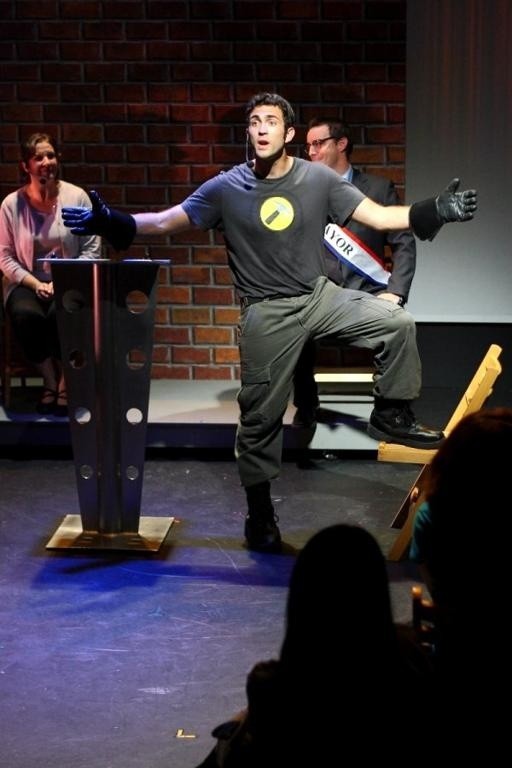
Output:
[293,406,315,425]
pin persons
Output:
[1,130,105,417]
[405,404,512,680]
[215,522,488,767]
[58,89,480,553]
[284,113,419,466]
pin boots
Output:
[243,505,281,556]
[367,400,446,449]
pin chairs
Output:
[408,583,440,676]
[377,344,502,561]
[0,300,90,409]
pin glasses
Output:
[304,136,337,150]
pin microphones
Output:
[245,131,255,168]
[27,173,46,186]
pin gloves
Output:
[408,178,477,242]
[61,190,136,251]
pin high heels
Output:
[37,386,71,416]
